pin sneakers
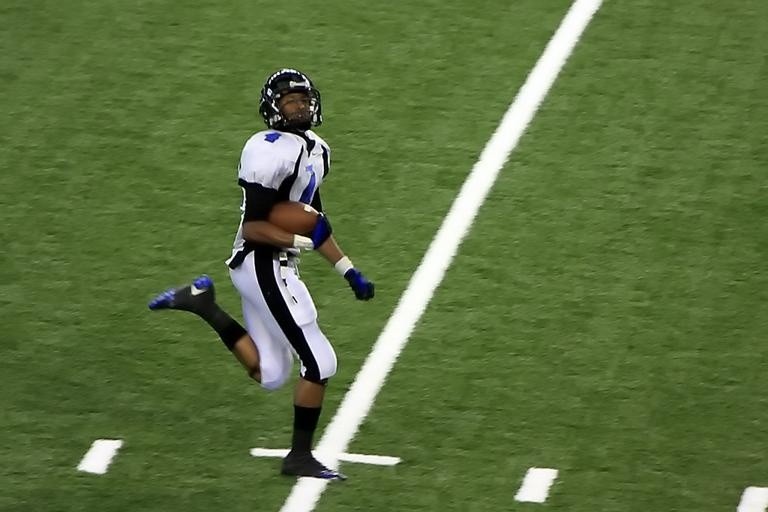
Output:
[149,275,215,312]
[280,456,347,481]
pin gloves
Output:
[335,255,375,300]
[294,211,332,252]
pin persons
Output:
[146,64,375,482]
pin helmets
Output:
[259,69,323,131]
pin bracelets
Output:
[293,232,315,252]
[334,255,355,276]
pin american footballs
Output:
[267,201,320,235]
